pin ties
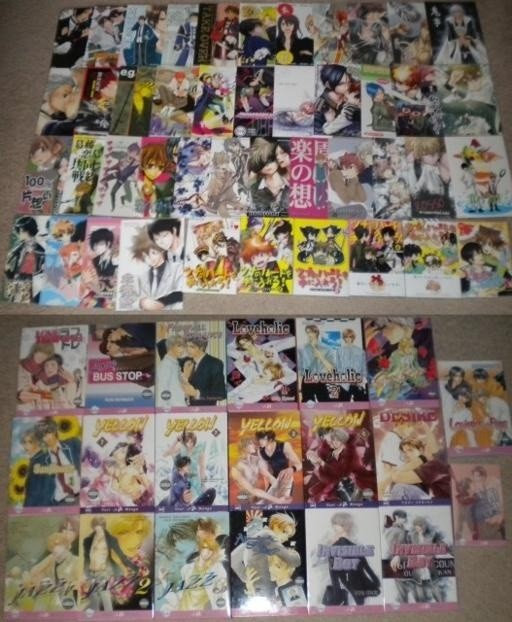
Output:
[54,559,63,586]
[48,446,75,495]
[151,269,160,297]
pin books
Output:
[1,1,512,311]
[4,317,512,618]
